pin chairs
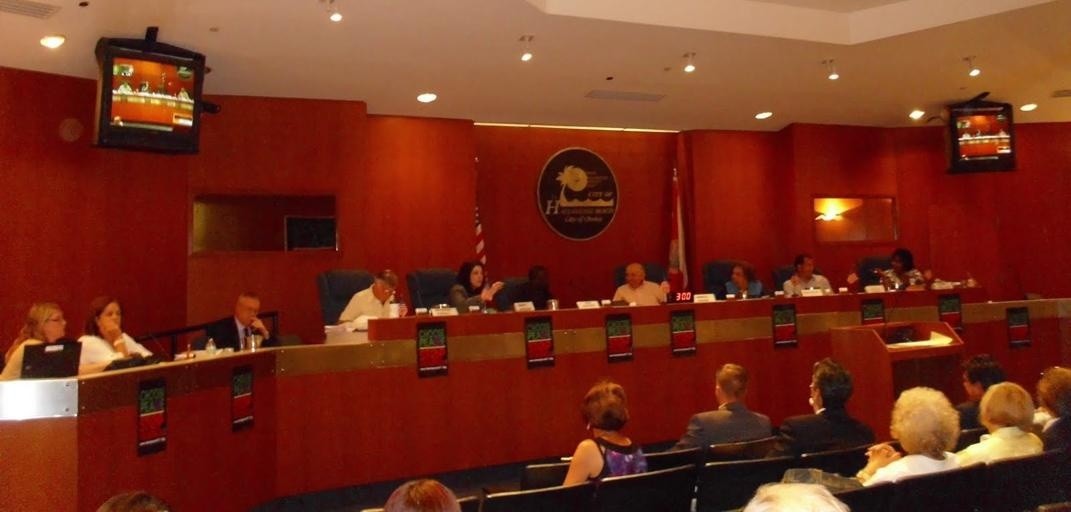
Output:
[316,269,374,325]
[772,268,820,291]
[495,281,533,310]
[703,260,752,297]
[615,265,665,289]
[407,270,458,311]
[858,259,892,292]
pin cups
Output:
[552,299,557,311]
[249,334,256,352]
[390,301,400,318]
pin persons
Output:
[95,490,172,512]
[611,262,667,307]
[206,292,275,353]
[383,480,463,512]
[668,363,770,452]
[783,254,833,296]
[446,260,504,314]
[504,265,554,310]
[0,302,67,381]
[962,129,1007,139]
[741,356,1071,512]
[334,269,408,332]
[869,248,924,290]
[563,383,648,486]
[716,263,764,300]
[75,297,154,376]
[118,80,189,101]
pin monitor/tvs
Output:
[19,341,82,380]
[941,103,1017,173]
[91,42,204,155]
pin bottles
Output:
[206,338,216,355]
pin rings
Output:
[874,271,877,273]
